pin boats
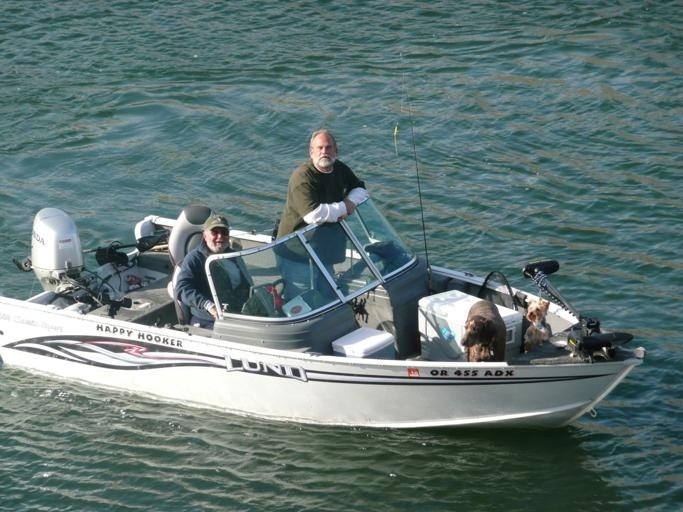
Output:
[0,190,645,436]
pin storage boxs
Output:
[330,326,395,360]
[416,289,522,361]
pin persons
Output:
[270,128,370,317]
[172,215,254,331]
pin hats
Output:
[202,215,229,230]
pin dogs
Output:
[522,299,553,355]
[460,300,508,368]
